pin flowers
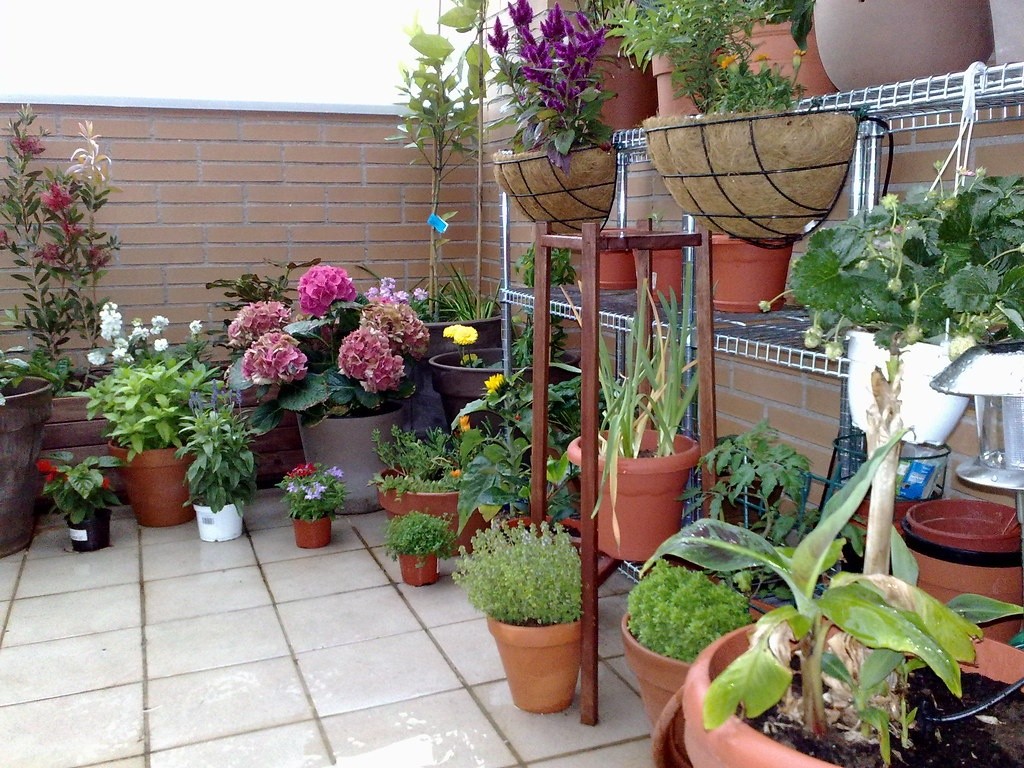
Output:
[488,0,626,165]
[276,463,351,517]
[36,446,124,523]
[606,0,820,117]
[0,102,120,396]
[442,323,507,481]
[228,267,431,436]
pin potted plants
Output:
[91,162,1024,766]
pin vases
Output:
[298,397,401,515]
[0,373,57,559]
[587,1,1023,312]
[289,512,334,547]
[63,509,113,552]
